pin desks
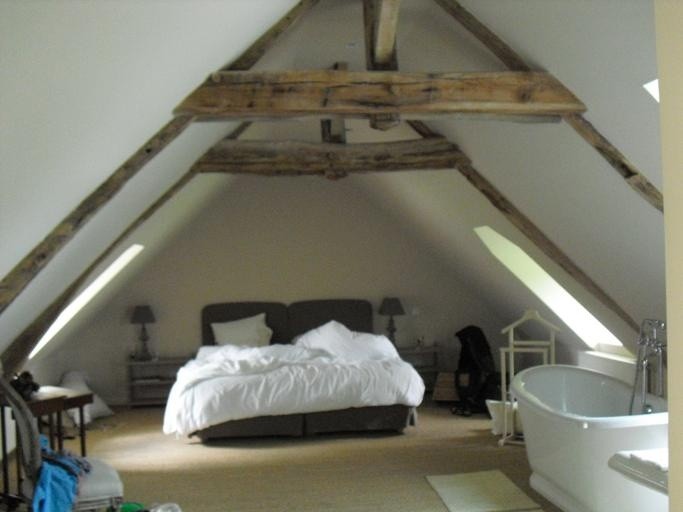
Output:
[37,385,93,457]
[0,387,66,504]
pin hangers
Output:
[501,305,561,334]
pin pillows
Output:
[56,369,113,425]
[210,311,273,349]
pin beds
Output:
[192,300,305,441]
[288,299,416,435]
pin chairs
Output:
[0,370,124,512]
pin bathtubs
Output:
[512,364,669,512]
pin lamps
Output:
[378,297,405,351]
[130,306,155,361]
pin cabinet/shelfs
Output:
[601,464,669,511]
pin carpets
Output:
[425,468,541,512]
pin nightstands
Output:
[398,345,456,392]
[126,353,195,411]
